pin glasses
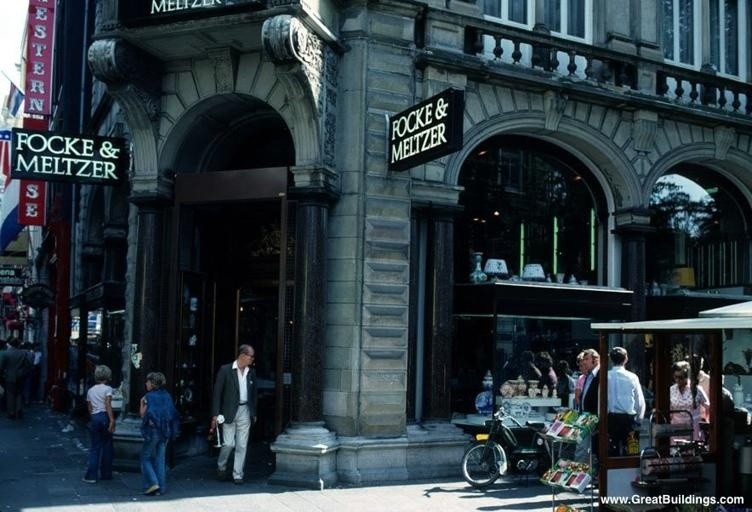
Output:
[250,354,255,358]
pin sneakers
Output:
[81,476,98,485]
[217,468,227,482]
[233,476,244,486]
[143,484,161,496]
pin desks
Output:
[451,394,564,475]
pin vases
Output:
[468,250,488,286]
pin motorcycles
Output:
[460,406,598,489]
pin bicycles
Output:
[44,371,77,419]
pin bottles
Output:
[744,393,751,406]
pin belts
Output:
[238,401,249,407]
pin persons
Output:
[0,336,42,420]
[515,350,575,408]
[575,347,710,489]
[138,372,180,496]
[82,365,116,484]
[211,344,258,484]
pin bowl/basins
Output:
[502,399,558,423]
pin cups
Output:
[556,272,565,282]
